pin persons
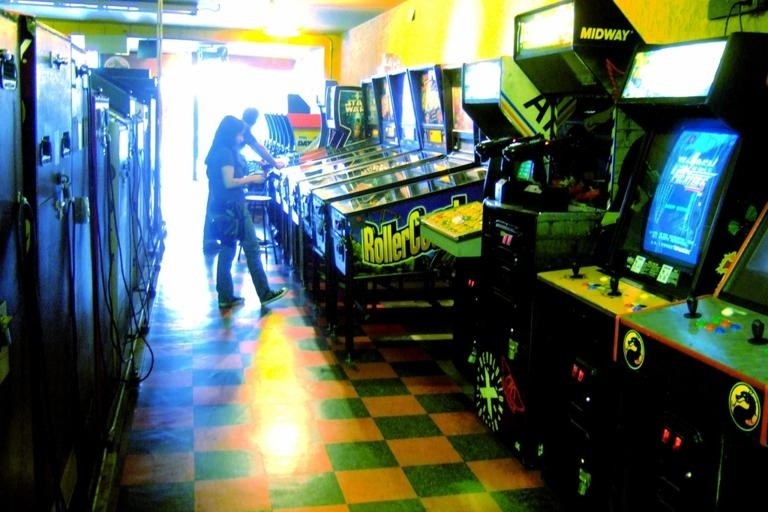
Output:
[204,108,286,257]
[204,115,289,308]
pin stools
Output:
[237,194,278,266]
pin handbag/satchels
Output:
[208,201,246,242]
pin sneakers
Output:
[220,296,245,307]
[261,288,288,305]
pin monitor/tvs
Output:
[638,122,740,269]
[516,159,534,181]
[745,219,768,274]
